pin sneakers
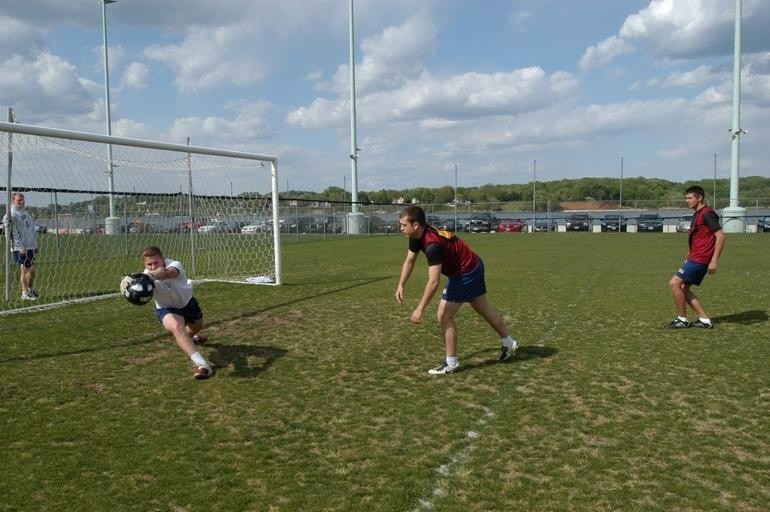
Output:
[499,337,520,362]
[20,289,36,301]
[194,364,213,379]
[428,360,463,375]
[664,318,689,330]
[192,333,207,345]
[28,287,39,299]
[690,319,714,329]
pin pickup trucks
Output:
[465,213,502,232]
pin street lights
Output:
[97,0,123,235]
[133,184,136,203]
[286,176,290,200]
[713,152,718,210]
[619,155,624,231]
[230,181,233,194]
[533,159,536,232]
[454,163,459,233]
[343,175,346,213]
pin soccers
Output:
[125,276,154,304]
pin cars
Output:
[600,214,628,232]
[676,214,693,232]
[0,212,462,235]
[565,214,593,232]
[497,219,528,232]
[534,218,558,232]
[636,213,664,232]
[758,217,770,231]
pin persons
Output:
[395,207,519,375]
[664,185,726,329]
[121,246,213,380]
[2,192,46,302]
